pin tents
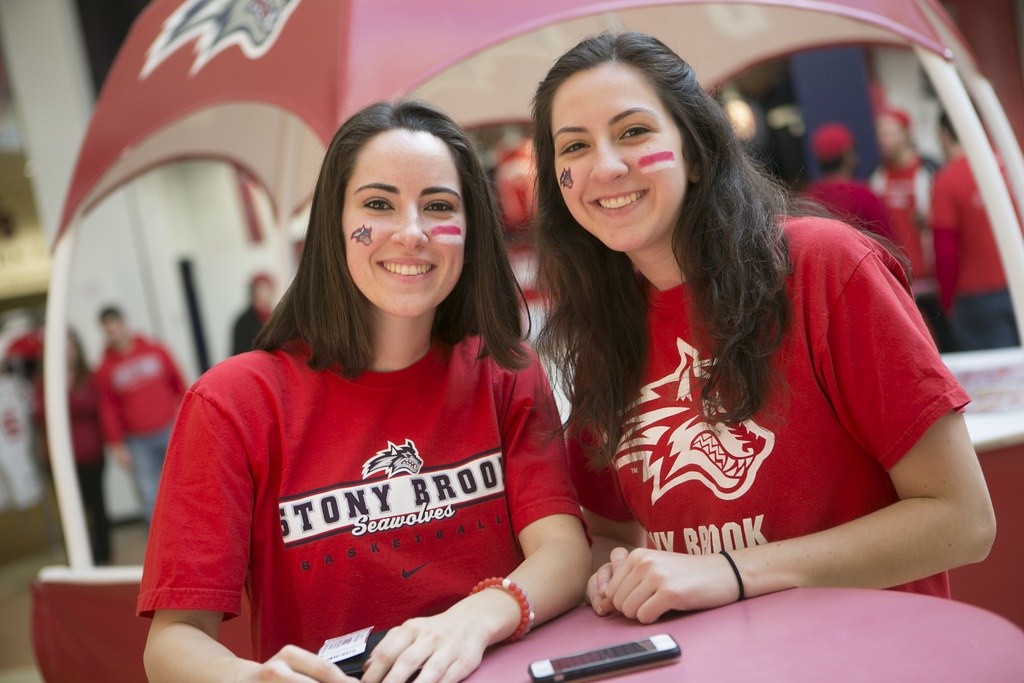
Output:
[43,1,1024,573]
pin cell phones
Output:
[529,633,682,683]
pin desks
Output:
[464,586,1023,683]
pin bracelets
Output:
[470,577,535,644]
[720,550,744,601]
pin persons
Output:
[137,102,594,683]
[233,275,275,357]
[791,108,1024,350]
[8,327,111,566]
[529,32,996,623]
[96,305,187,525]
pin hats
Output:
[813,123,854,163]
